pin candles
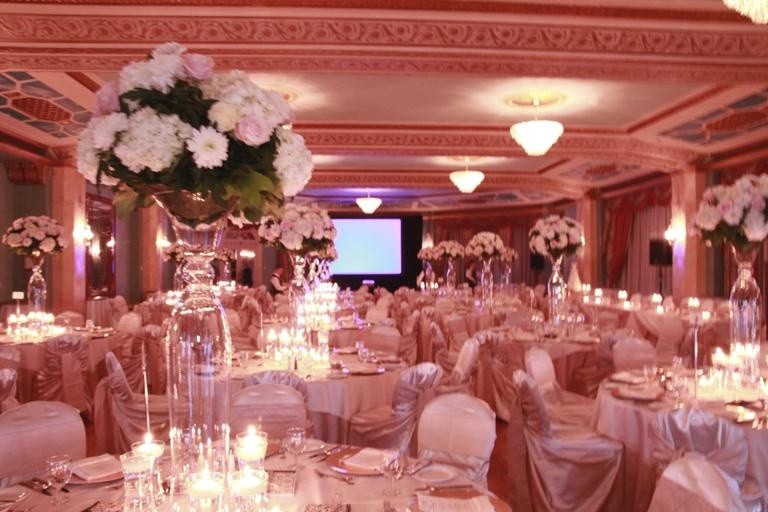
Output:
[698,369,714,395]
[298,284,339,324]
[719,371,740,396]
[236,425,265,473]
[185,461,224,512]
[710,346,727,372]
[257,328,330,369]
[227,464,267,512]
[7,309,62,339]
[759,377,767,397]
[133,431,165,493]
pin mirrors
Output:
[85,192,116,303]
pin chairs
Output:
[347,362,444,458]
[359,285,369,296]
[0,342,23,415]
[643,409,762,511]
[516,288,544,325]
[127,325,166,392]
[358,325,401,358]
[227,383,307,450]
[400,305,422,362]
[112,295,133,332]
[371,294,394,322]
[96,351,170,454]
[612,337,656,375]
[512,370,623,512]
[54,312,84,330]
[116,313,141,344]
[524,346,595,424]
[425,321,449,364]
[475,329,524,423]
[37,333,94,424]
[247,297,266,323]
[416,393,498,491]
[0,401,88,486]
[443,312,470,348]
[443,339,480,389]
[395,286,413,304]
[625,311,684,370]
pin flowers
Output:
[692,174,768,259]
[466,231,506,265]
[3,215,66,260]
[417,247,439,263]
[528,214,585,259]
[257,202,337,256]
[76,41,317,227]
[217,243,237,266]
[497,247,519,263]
[435,240,466,261]
[162,240,189,265]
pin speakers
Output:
[531,254,544,270]
[649,238,672,266]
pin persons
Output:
[464,261,477,287]
[269,266,290,302]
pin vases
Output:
[730,243,763,378]
[224,259,231,283]
[173,262,184,292]
[26,253,48,319]
[289,251,308,314]
[425,259,435,291]
[481,258,493,306]
[548,255,567,327]
[152,192,240,489]
[504,261,512,286]
[447,256,456,297]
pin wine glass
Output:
[439,277,729,342]
[643,356,768,412]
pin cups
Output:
[257,281,339,379]
[6,312,54,342]
[119,430,270,512]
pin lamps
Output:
[664,222,680,246]
[449,157,485,194]
[73,219,92,244]
[511,93,565,157]
[356,190,382,215]
[90,244,101,259]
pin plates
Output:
[0,438,479,512]
[192,346,386,379]
